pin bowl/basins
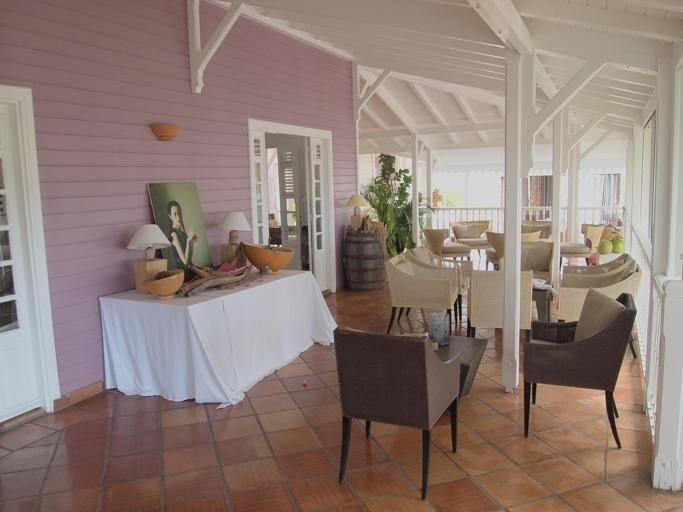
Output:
[144,269,185,299]
[240,240,293,274]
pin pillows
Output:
[574,287,627,343]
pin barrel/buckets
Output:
[343,232,387,290]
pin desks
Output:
[96,267,338,409]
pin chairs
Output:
[383,220,641,360]
[329,325,462,500]
[522,291,639,449]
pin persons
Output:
[166,201,196,282]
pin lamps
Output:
[126,224,172,292]
[216,212,251,264]
[344,193,369,229]
